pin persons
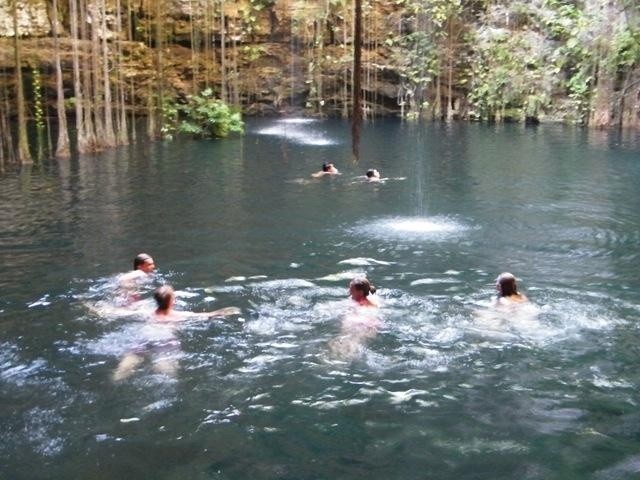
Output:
[152,284,241,321]
[496,272,527,304]
[366,168,380,181]
[348,279,378,309]
[314,162,338,177]
[134,253,155,274]
